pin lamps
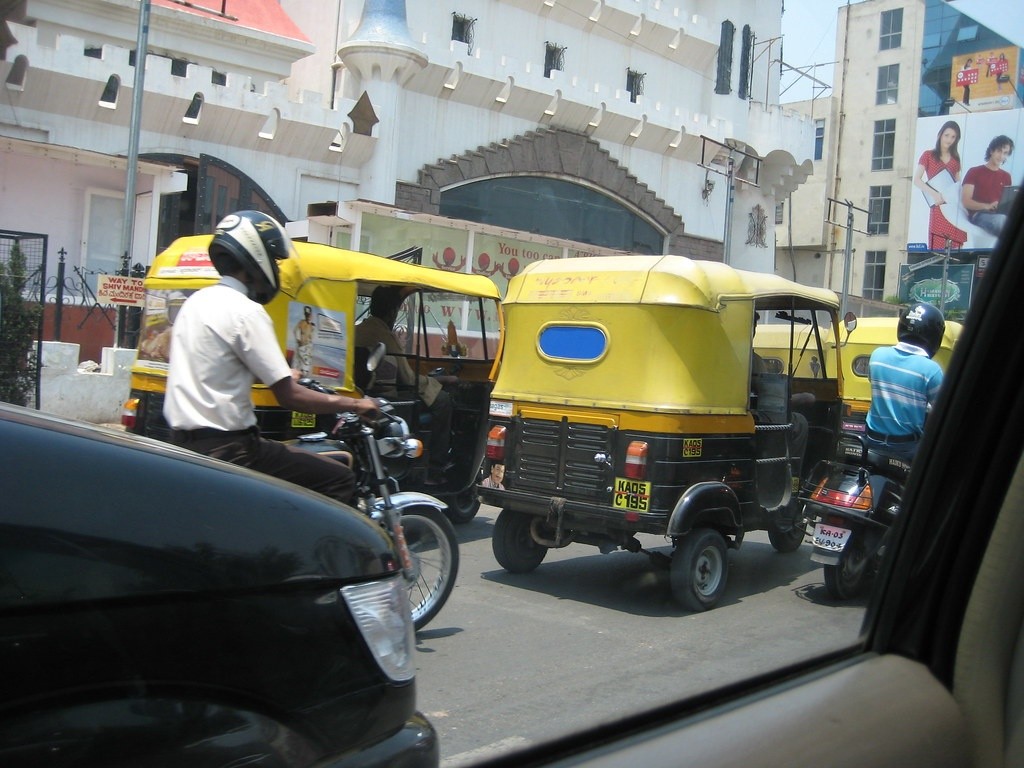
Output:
[944,98,971,113]
[996,74,1024,107]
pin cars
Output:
[1,404,441,768]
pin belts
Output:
[866,427,920,443]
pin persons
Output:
[751,311,810,490]
[354,284,457,474]
[867,303,947,463]
[915,119,1017,253]
[294,303,315,378]
[163,210,382,506]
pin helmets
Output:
[897,304,945,359]
[208,209,290,305]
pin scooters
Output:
[795,353,932,603]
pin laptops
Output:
[981,185,1020,214]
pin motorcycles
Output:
[257,373,461,633]
[121,234,508,528]
[469,254,857,615]
[750,314,964,439]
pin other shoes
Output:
[424,475,450,485]
